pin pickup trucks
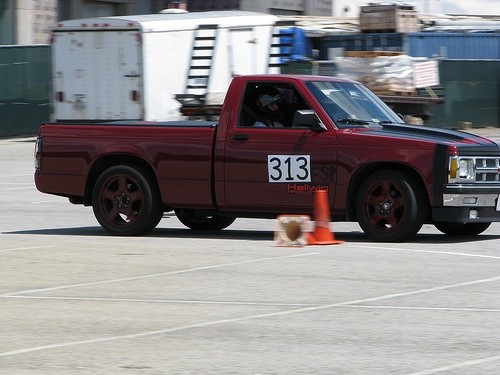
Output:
[33,72,500,242]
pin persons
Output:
[249,84,285,128]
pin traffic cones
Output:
[273,215,309,247]
[307,188,344,245]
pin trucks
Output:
[47,9,314,122]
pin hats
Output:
[257,94,280,106]
